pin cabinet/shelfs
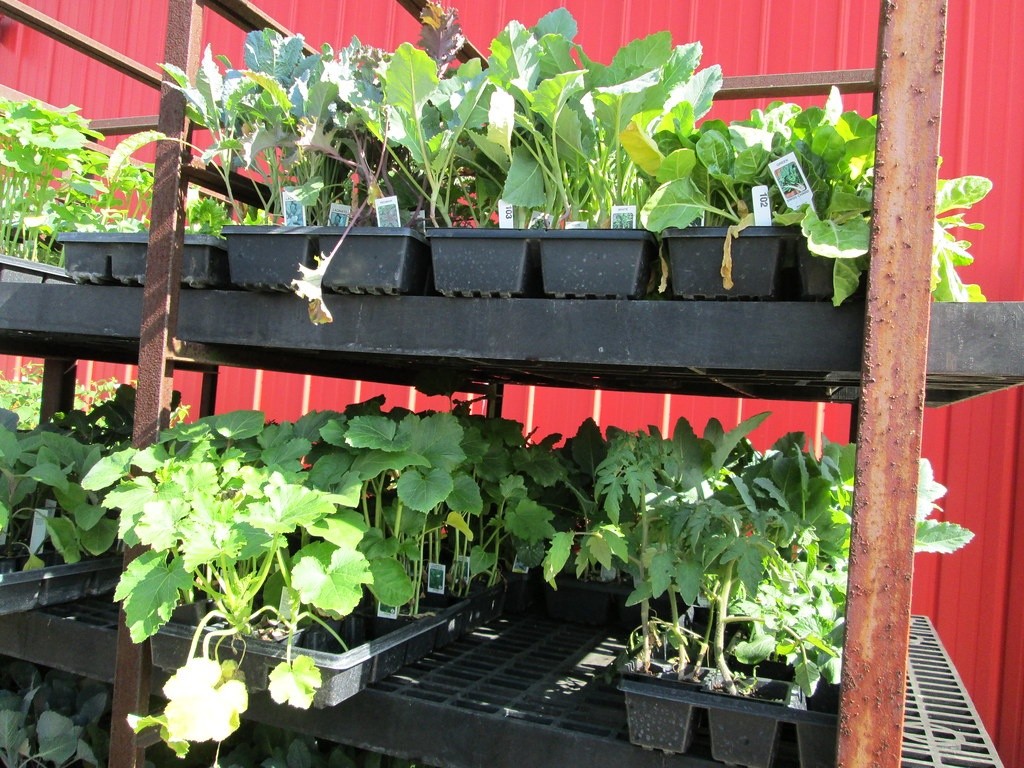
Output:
[0,0,956,768]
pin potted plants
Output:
[0,0,994,320]
[0,383,976,768]
